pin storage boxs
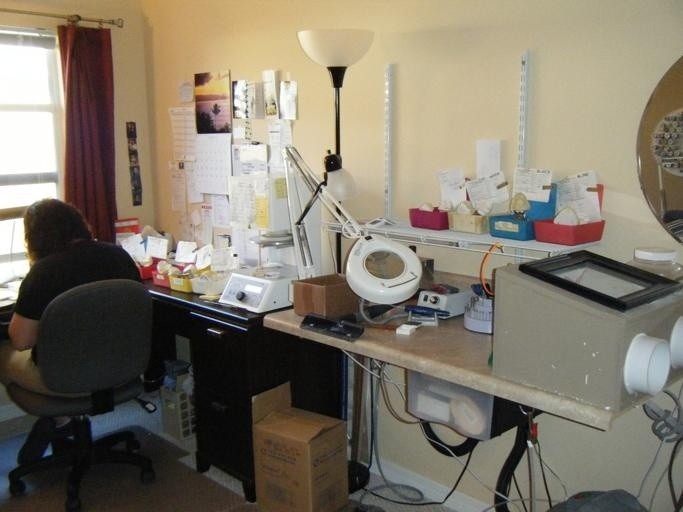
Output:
[252,381,347,512]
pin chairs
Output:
[6,279,157,512]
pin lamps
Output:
[284,28,377,314]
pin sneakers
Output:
[17,415,79,465]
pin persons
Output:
[0,197,143,398]
